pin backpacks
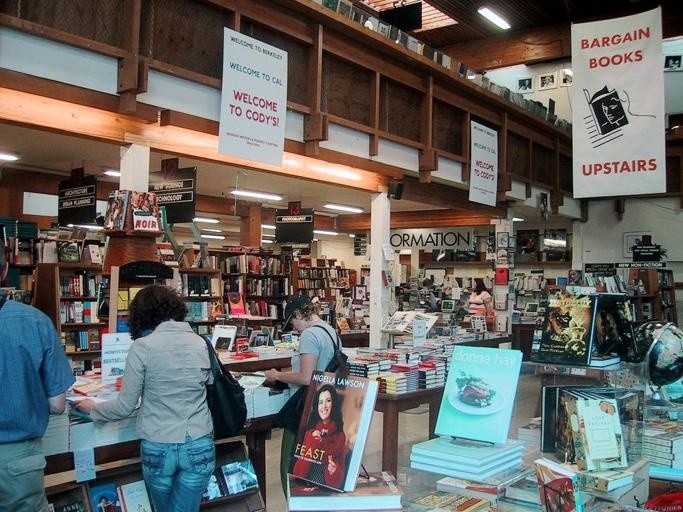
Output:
[199,333,248,438]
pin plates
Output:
[447,382,505,416]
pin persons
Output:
[0,242,77,512]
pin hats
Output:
[282,300,308,332]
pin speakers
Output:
[387,181,404,200]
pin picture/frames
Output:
[662,53,683,73]
[517,68,572,95]
[624,231,653,258]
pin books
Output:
[433,270,677,444]
[77,371,682,511]
[2,217,447,392]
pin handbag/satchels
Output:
[271,323,349,430]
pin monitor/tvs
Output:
[441,300,455,312]
[525,302,539,316]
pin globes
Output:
[627,321,683,408]
